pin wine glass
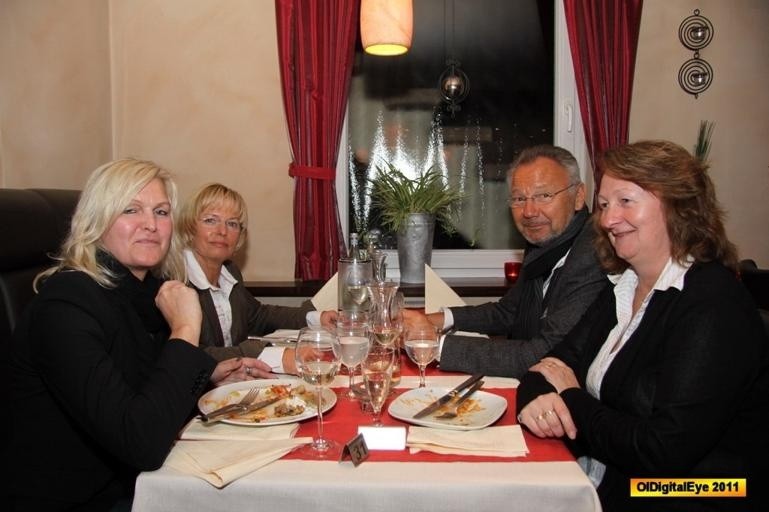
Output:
[295,252,440,460]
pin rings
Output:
[544,409,554,417]
[548,363,554,368]
[245,368,252,375]
[534,414,544,421]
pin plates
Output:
[389,385,510,432]
[194,377,335,426]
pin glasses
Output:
[503,182,577,209]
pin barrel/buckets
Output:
[338,259,377,312]
[395,212,435,284]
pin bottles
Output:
[343,230,384,261]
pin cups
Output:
[505,259,523,283]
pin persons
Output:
[375,146,617,378]
[2,158,271,512]
[150,184,350,377]
[515,141,769,511]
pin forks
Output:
[434,378,487,420]
[194,385,258,420]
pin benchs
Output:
[0,187,85,357]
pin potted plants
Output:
[366,156,471,284]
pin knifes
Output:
[200,395,287,425]
[412,365,485,421]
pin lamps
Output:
[359,0,415,56]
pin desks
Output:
[236,278,519,303]
